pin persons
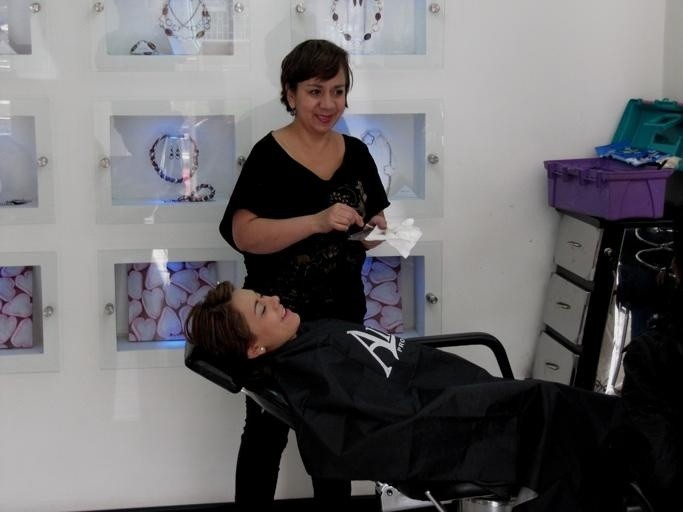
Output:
[185,283,683,509]
[217,38,388,508]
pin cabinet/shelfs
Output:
[529,214,633,396]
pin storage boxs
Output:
[542,97,683,221]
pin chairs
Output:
[186,327,534,512]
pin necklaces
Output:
[331,0,385,52]
[362,127,394,199]
[148,131,205,184]
[159,1,213,41]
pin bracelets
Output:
[130,36,159,57]
[164,184,217,203]
